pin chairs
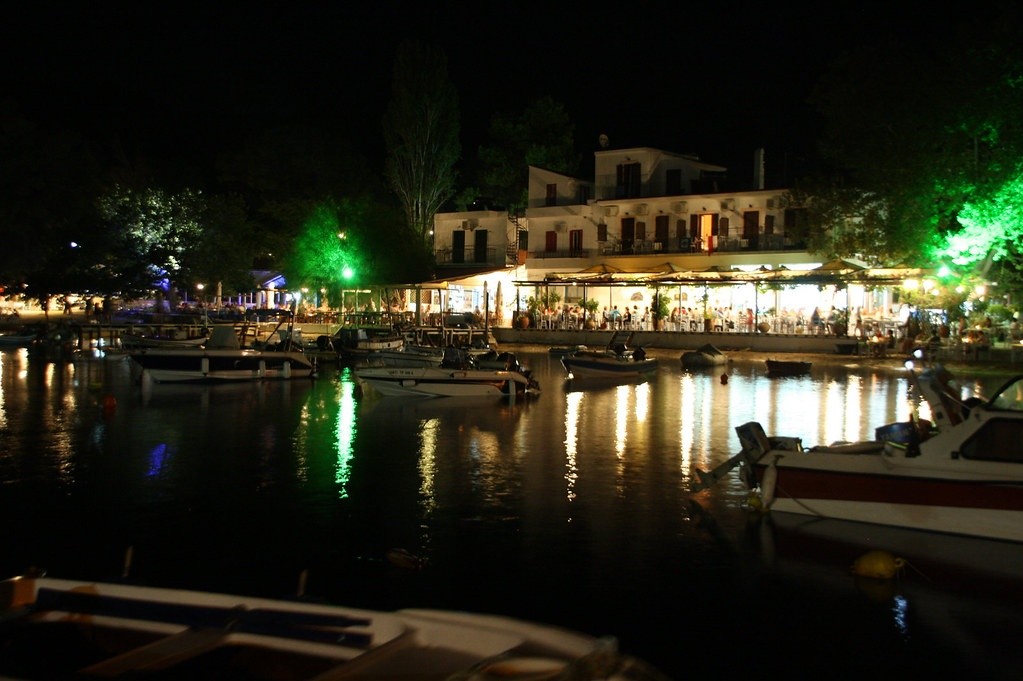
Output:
[536,311,834,332]
[854,318,995,365]
[597,239,654,255]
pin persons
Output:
[94,296,483,329]
[530,303,941,360]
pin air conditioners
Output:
[637,197,780,215]
[462,220,473,229]
[555,223,568,233]
[604,206,619,217]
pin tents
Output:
[517,263,934,338]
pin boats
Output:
[0,308,540,400]
[689,360,1023,546]
[909,360,1023,463]
[558,341,661,382]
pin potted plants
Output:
[517,313,530,329]
[831,307,849,335]
[584,298,599,330]
[648,290,671,331]
[702,309,715,332]
